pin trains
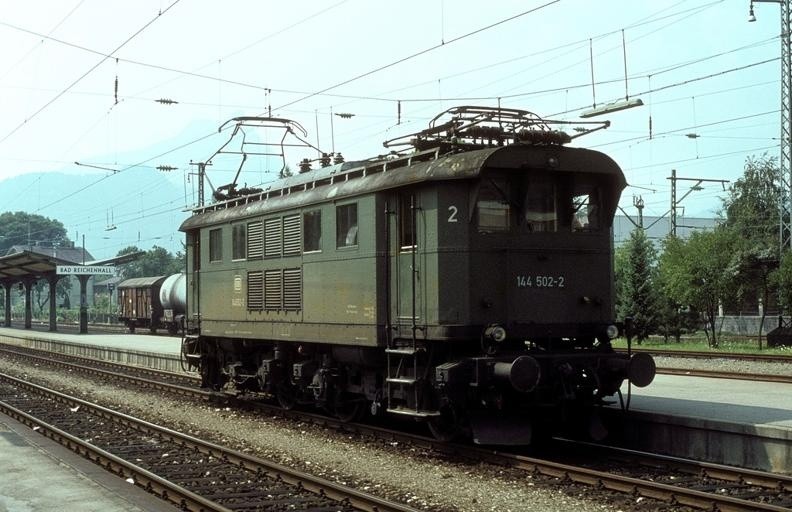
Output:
[117,274,188,335]
[179,106,658,453]
